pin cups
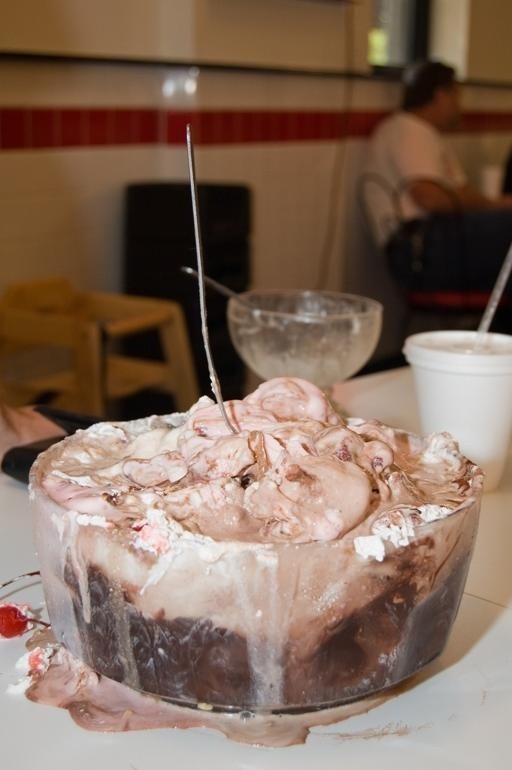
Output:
[403,329,512,494]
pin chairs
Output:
[350,165,512,364]
[1,273,197,442]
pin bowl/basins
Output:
[25,400,484,716]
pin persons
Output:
[357,55,510,333]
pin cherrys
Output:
[0,605,49,639]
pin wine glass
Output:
[227,288,383,424]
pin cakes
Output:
[7,378,484,749]
